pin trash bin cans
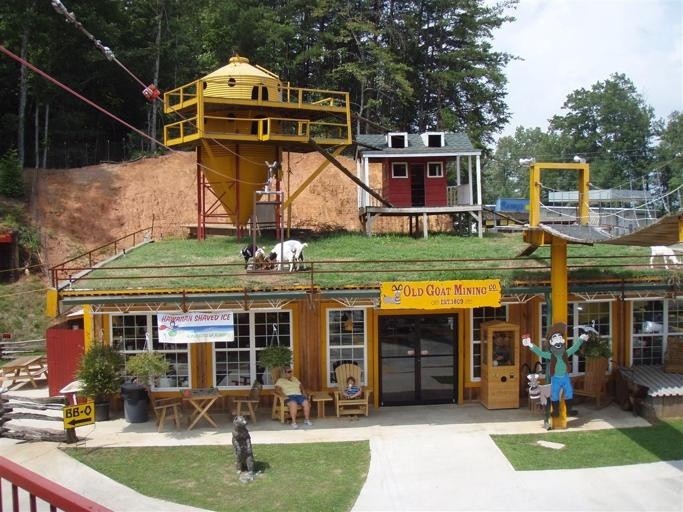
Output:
[121,383,148,423]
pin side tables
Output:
[312,393,333,418]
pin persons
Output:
[273,368,314,430]
[522,326,599,419]
[341,376,362,422]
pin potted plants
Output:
[73,338,170,424]
[257,344,292,385]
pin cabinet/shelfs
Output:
[479,319,522,411]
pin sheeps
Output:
[649,245,682,270]
[240,238,309,274]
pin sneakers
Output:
[291,422,299,428]
[304,419,313,426]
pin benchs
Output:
[0,365,47,389]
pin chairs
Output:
[226,379,263,425]
[146,389,182,432]
[570,354,615,410]
[330,363,371,419]
[268,364,314,423]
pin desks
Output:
[180,389,222,432]
[0,355,47,387]
